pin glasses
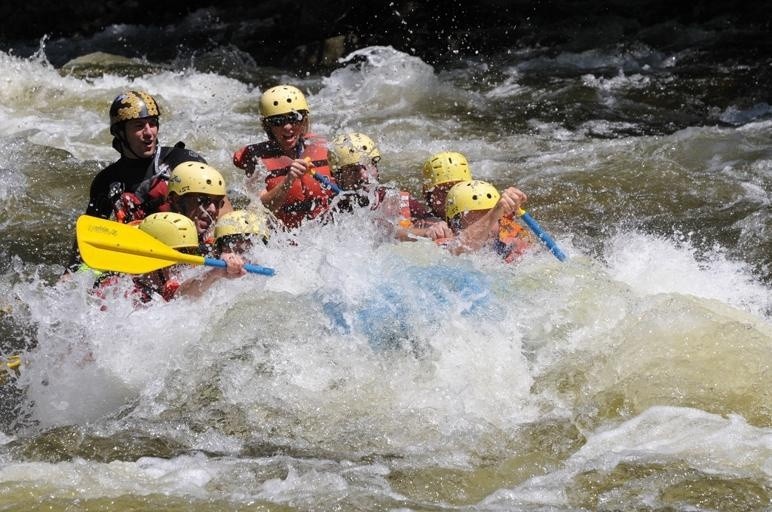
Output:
[268,113,304,126]
[191,194,225,209]
[126,117,157,130]
[221,236,250,248]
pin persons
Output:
[92,212,248,308]
[215,210,267,256]
[65,90,208,278]
[407,151,470,241]
[233,84,337,227]
[108,162,226,234]
[327,132,427,218]
[445,180,528,256]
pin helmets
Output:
[422,151,471,193]
[326,132,381,176]
[213,210,270,245]
[137,210,199,250]
[445,180,500,228]
[164,160,225,204]
[259,84,310,119]
[110,89,160,125]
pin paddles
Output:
[75,215,274,276]
